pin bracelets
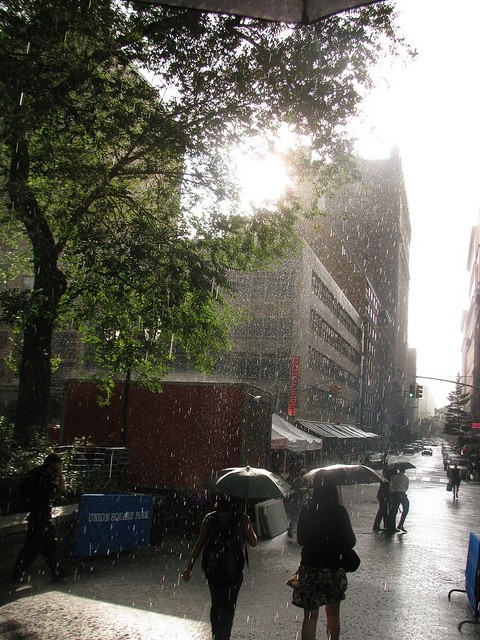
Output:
[58,473,63,477]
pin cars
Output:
[410,442,419,452]
[422,447,433,456]
[368,452,383,467]
[424,437,439,446]
[440,438,479,481]
[404,445,414,455]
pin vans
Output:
[414,440,424,449]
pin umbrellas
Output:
[300,463,388,505]
[209,465,292,514]
[383,461,416,473]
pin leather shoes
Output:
[394,529,400,532]
[397,525,407,533]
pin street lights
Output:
[102,315,162,447]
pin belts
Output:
[394,492,405,495]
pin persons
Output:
[373,468,391,532]
[11,455,71,585]
[387,468,409,532]
[449,463,460,501]
[286,486,361,640]
[182,494,257,639]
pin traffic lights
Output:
[416,385,423,398]
[408,383,414,398]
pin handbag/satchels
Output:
[345,549,361,573]
[447,483,452,491]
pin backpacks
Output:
[201,511,245,586]
